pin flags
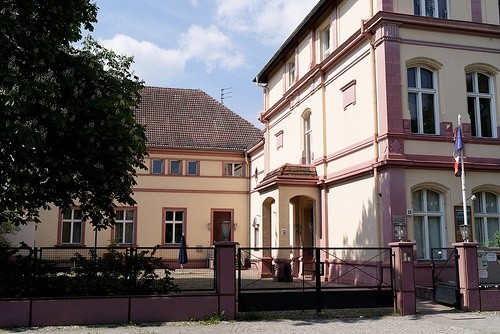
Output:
[452,117,465,178]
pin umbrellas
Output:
[179,235,188,269]
